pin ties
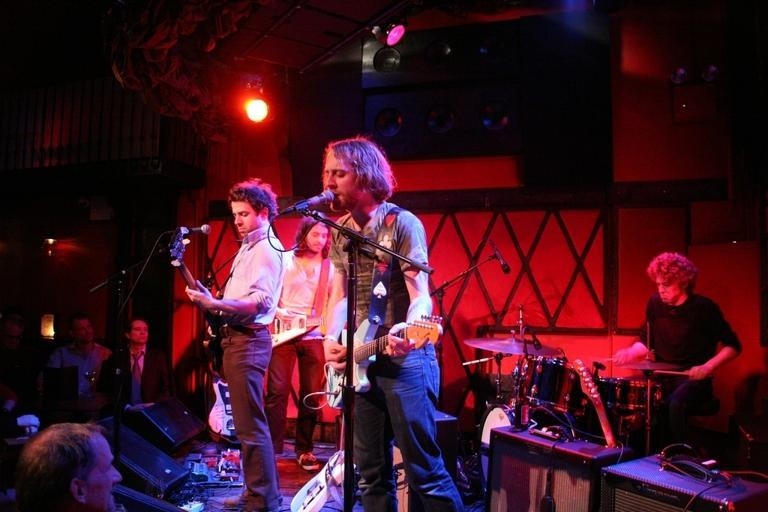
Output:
[131,352,143,406]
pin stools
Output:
[677,395,721,448]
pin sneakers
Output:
[224,489,256,506]
[297,450,320,470]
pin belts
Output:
[223,324,265,338]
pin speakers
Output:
[123,396,206,450]
[107,482,183,511]
[85,415,192,497]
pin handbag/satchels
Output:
[455,453,483,507]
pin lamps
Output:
[653,41,725,88]
[345,14,408,76]
[38,310,57,341]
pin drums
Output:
[595,377,664,412]
[512,356,587,418]
[475,400,517,497]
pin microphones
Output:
[175,223,211,235]
[487,236,511,274]
[280,189,334,215]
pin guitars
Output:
[170,231,227,383]
[204,320,237,437]
[290,450,344,511]
[267,309,322,348]
[327,315,444,408]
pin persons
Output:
[182,176,287,511]
[96,316,173,417]
[609,252,741,459]
[314,135,465,510]
[256,210,345,474]
[0,310,50,441]
[44,313,113,414]
[11,419,124,511]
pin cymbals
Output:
[464,336,565,359]
[614,357,682,370]
